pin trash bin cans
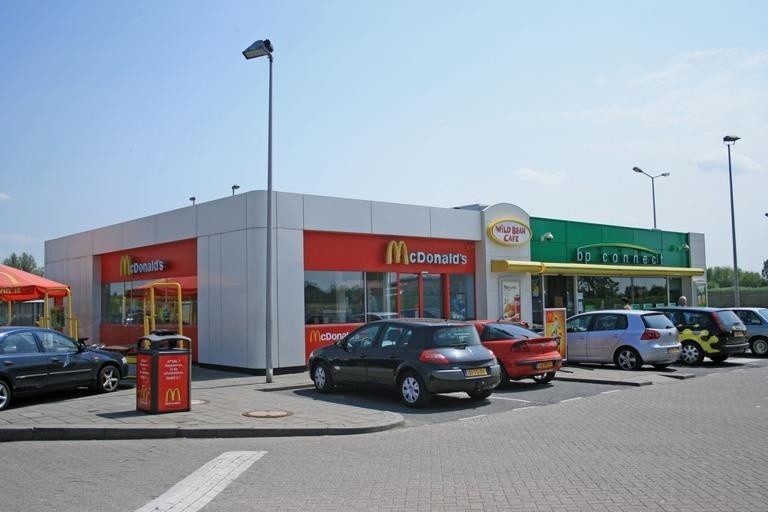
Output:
[136,329,192,414]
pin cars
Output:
[442,320,563,389]
[694,306,767,357]
[0,325,129,412]
[642,308,750,363]
[110,309,189,325]
[536,309,684,372]
[305,318,504,408]
[400,308,462,319]
[352,312,405,320]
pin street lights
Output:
[231,184,239,196]
[720,135,740,308]
[632,167,670,229]
[188,197,196,207]
[236,37,279,385]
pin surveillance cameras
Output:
[684,245,689,251]
[547,234,553,240]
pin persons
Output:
[621,296,633,310]
[677,295,688,308]
